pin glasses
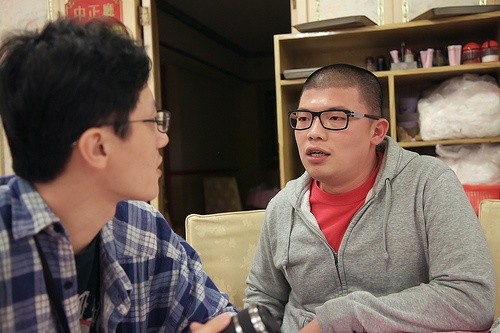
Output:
[287,109,381,131]
[87,110,171,133]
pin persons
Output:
[242,63,496,333]
[0,14,238,333]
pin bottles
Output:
[433,49,447,65]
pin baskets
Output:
[463,185,500,217]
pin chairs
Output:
[478,198,500,320]
[186,208,265,311]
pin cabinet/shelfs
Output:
[273,12,500,189]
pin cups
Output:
[420,49,434,67]
[447,45,462,66]
